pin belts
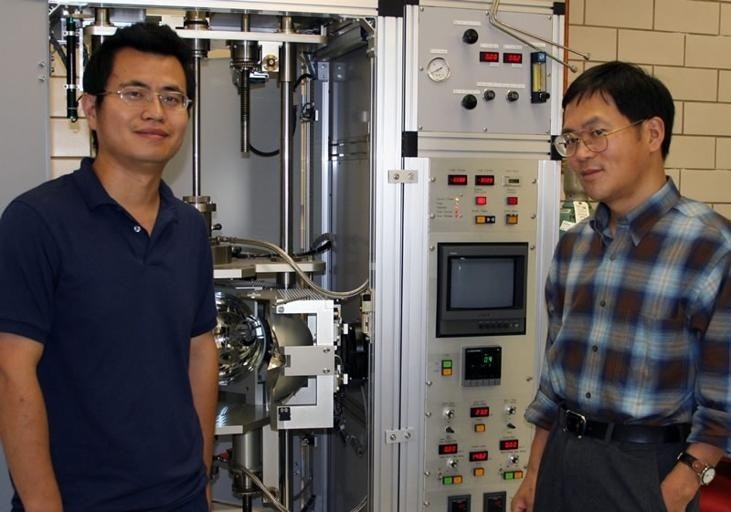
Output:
[555,409,677,447]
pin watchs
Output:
[677,449,718,487]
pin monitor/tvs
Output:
[436,242,528,337]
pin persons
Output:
[0,20,223,511]
[510,59,731,512]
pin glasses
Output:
[79,86,193,115]
[551,118,645,161]
